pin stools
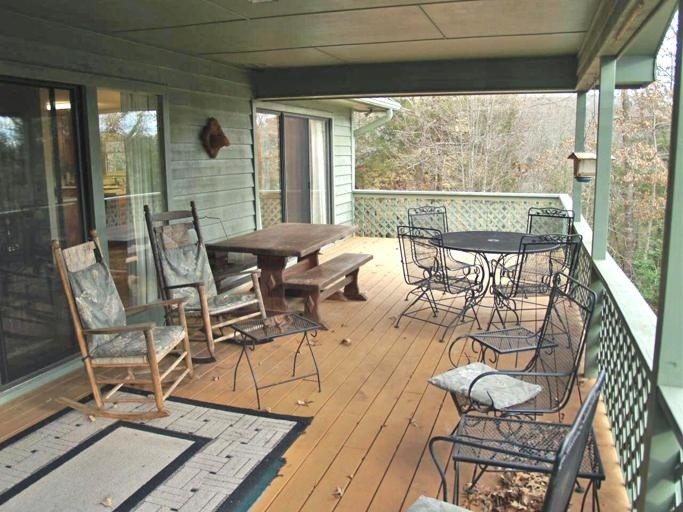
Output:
[229,314,321,412]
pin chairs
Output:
[52,227,190,421]
[143,201,274,364]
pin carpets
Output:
[0,385,315,512]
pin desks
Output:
[205,222,357,316]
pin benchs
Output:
[207,253,259,296]
[283,252,373,330]
